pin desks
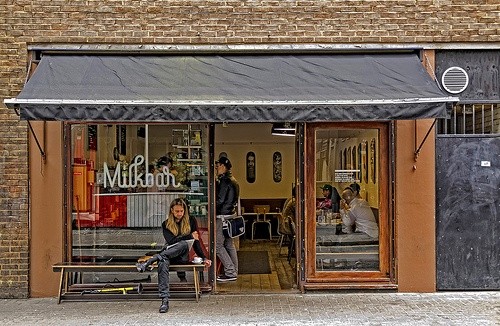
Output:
[243,210,347,237]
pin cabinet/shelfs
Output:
[172,128,208,215]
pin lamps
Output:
[271,122,296,137]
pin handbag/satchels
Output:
[222,215,245,238]
[138,256,160,265]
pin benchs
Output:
[52,226,209,305]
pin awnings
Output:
[2,53,460,162]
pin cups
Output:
[194,257,202,262]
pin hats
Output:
[320,184,332,190]
[215,156,232,169]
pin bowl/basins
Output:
[319,223,328,226]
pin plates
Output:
[191,261,204,264]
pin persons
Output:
[279,183,379,238]
[205,156,240,281]
[136,198,212,313]
[118,154,174,193]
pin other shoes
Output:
[217,274,238,282]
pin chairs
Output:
[251,205,272,240]
[287,215,297,263]
[275,208,292,256]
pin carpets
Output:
[219,250,272,276]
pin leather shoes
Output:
[136,262,146,272]
[159,304,168,313]
